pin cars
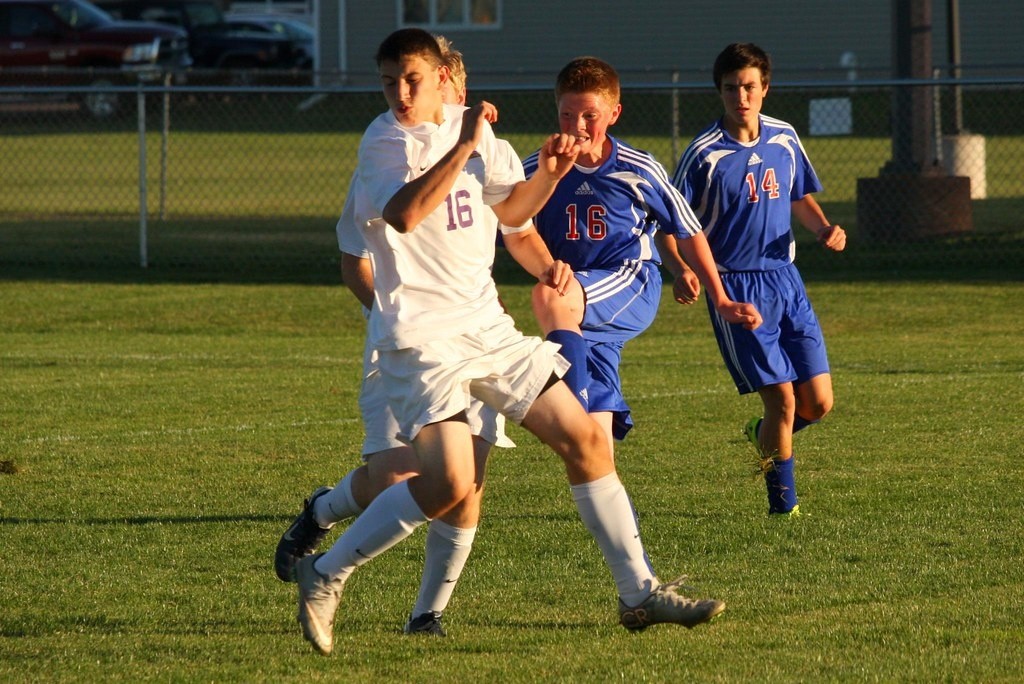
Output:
[88,0,223,42]
[0,0,192,121]
[185,15,314,90]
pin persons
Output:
[654,43,847,524]
[522,57,765,468]
[295,24,728,657]
[275,33,574,635]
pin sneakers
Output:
[275,486,337,582]
[744,415,764,457]
[618,574,726,634]
[292,552,344,657]
[769,506,811,523]
[404,611,445,637]
